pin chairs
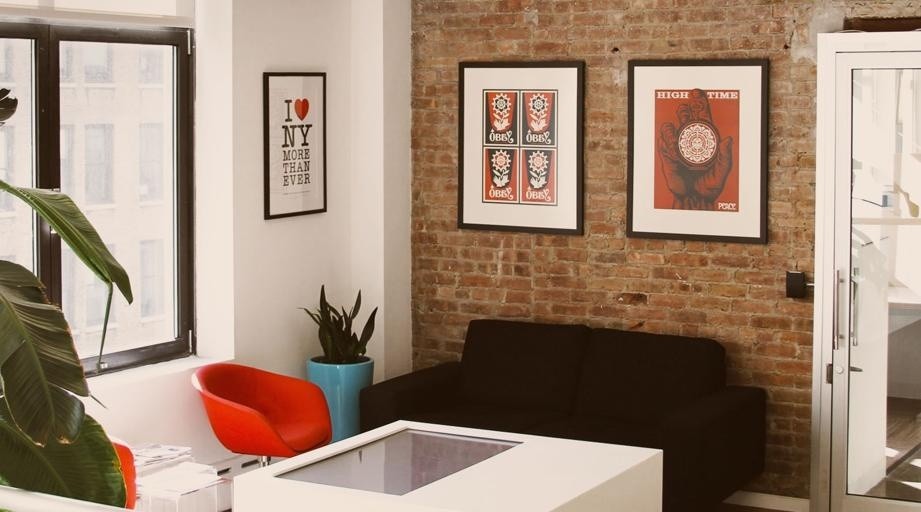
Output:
[190,359,333,469]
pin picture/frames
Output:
[260,69,330,222]
[453,55,774,247]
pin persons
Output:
[657,88,733,210]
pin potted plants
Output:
[300,282,383,440]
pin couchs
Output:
[356,315,767,512]
